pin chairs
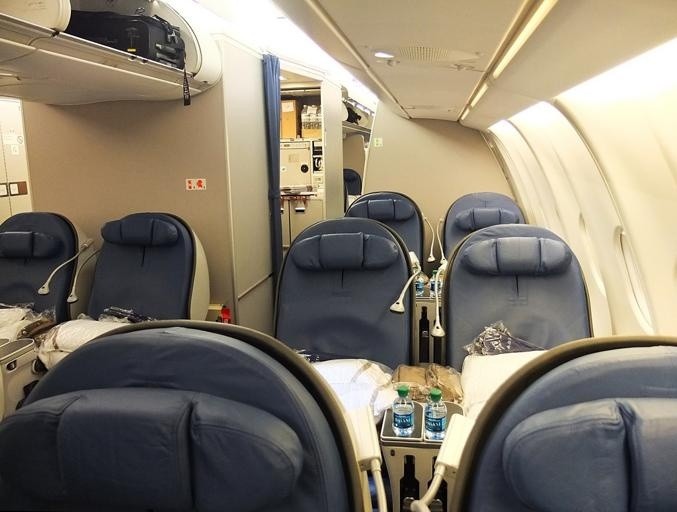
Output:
[0,167,677,512]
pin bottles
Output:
[391,385,415,436]
[423,388,448,440]
[428,270,442,297]
[414,270,425,298]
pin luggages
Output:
[64,8,187,71]
